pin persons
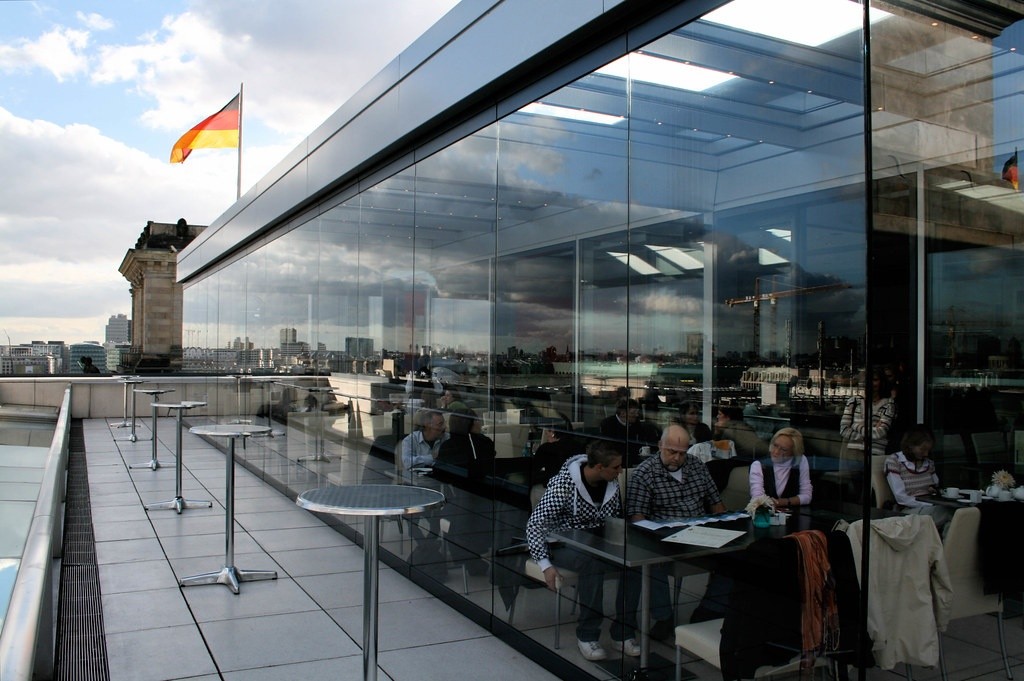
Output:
[79,356,100,373]
[401,366,950,660]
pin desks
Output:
[110,375,142,428]
[144,401,213,516]
[549,497,928,681]
[178,423,278,595]
[128,388,177,471]
[295,484,445,681]
[113,380,152,442]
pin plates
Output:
[942,495,964,499]
[960,490,977,494]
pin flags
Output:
[170,92,239,163]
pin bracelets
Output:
[785,497,791,506]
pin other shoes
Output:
[690,608,716,624]
[650,619,674,641]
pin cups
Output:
[1013,486,1024,500]
[970,491,982,503]
[999,491,1011,500]
[992,486,1002,498]
[944,488,959,497]
[639,446,650,455]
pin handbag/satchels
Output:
[406,540,449,583]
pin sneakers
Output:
[577,639,607,660]
[611,637,640,657]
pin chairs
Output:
[286,412,1024,681]
